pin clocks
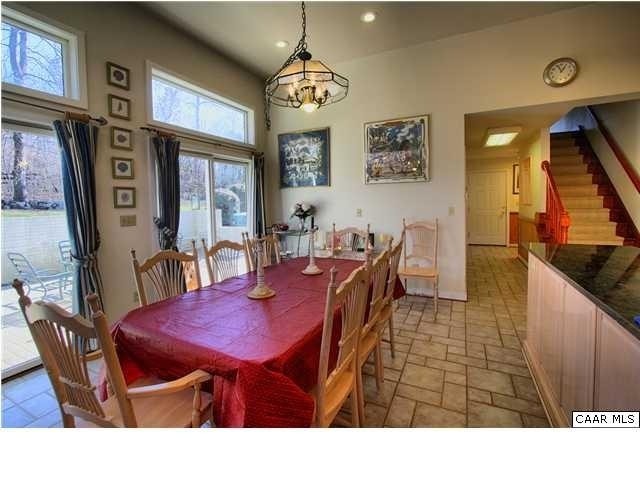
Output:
[540,55,582,88]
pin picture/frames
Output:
[112,186,136,210]
[276,125,334,192]
[110,125,135,152]
[512,162,520,197]
[105,58,133,91]
[112,156,136,181]
[108,93,133,123]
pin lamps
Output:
[481,123,523,150]
[263,1,349,121]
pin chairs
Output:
[4,207,454,428]
